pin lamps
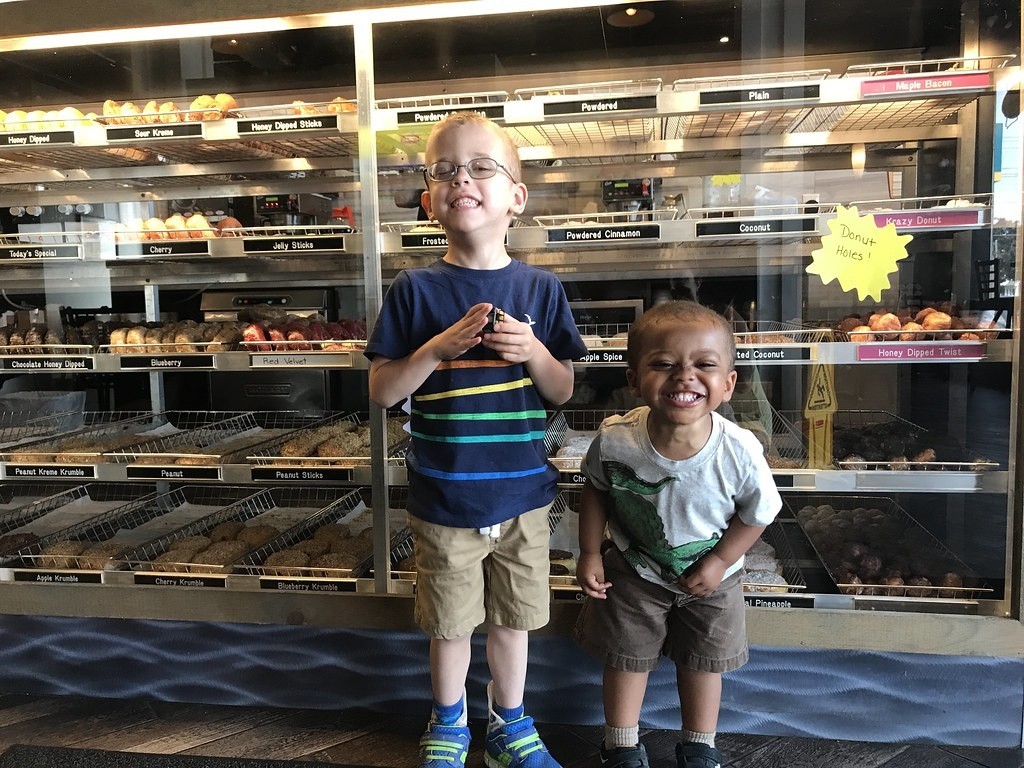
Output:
[607,7,655,27]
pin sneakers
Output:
[599,735,650,768]
[484,679,562,768]
[418,685,471,768]
[675,740,722,768]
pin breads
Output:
[110,212,244,240]
[0,93,356,135]
[0,307,368,358]
[0,418,411,584]
[540,302,998,593]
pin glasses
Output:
[423,158,516,191]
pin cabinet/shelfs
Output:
[0,0,1024,748]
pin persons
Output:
[572,300,782,768]
[364,113,587,768]
